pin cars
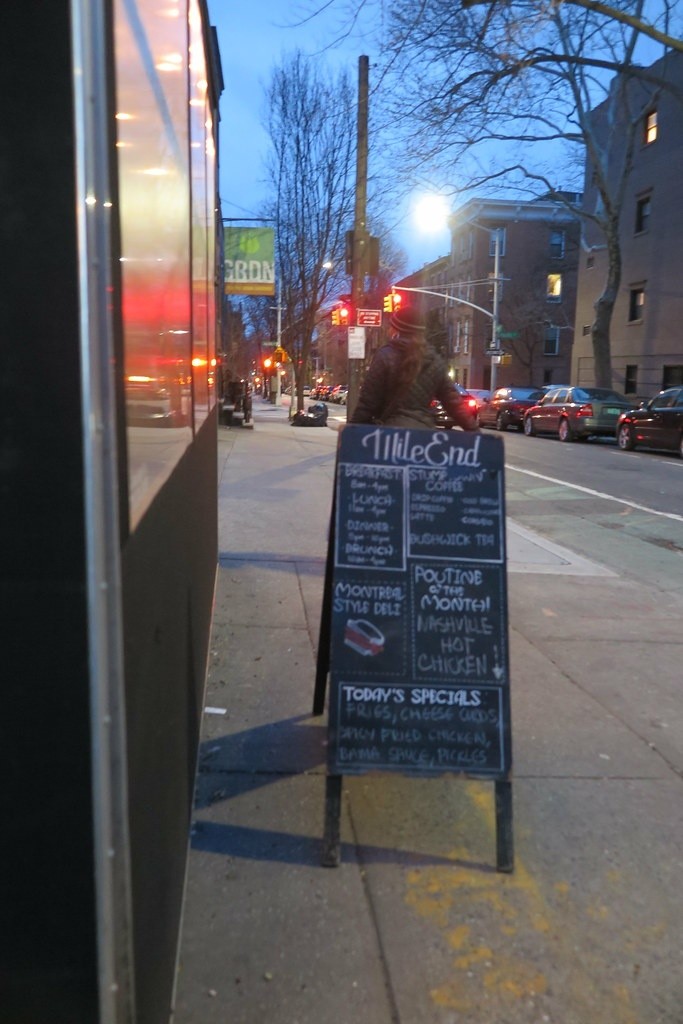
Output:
[286,383,347,404]
[430,383,477,429]
[464,389,489,424]
[476,385,545,431]
[523,386,641,442]
[615,385,683,459]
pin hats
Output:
[389,306,426,335]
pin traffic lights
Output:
[332,308,348,326]
[383,292,401,312]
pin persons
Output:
[223,369,243,412]
[350,306,480,431]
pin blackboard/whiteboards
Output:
[322,422,514,785]
